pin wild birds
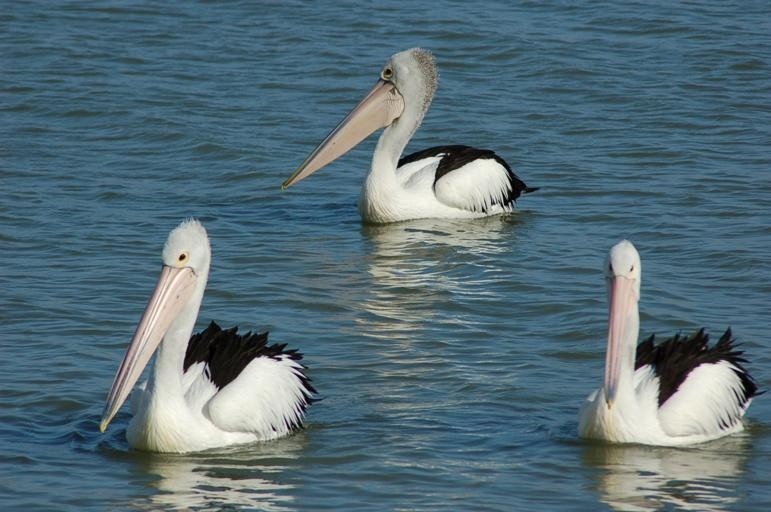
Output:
[95,212,326,454]
[576,237,768,451]
[279,44,543,226]
